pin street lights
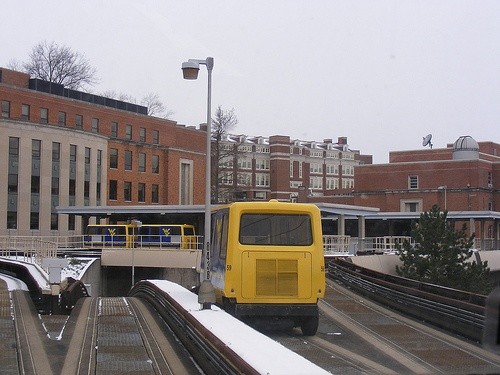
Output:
[181,56,216,310]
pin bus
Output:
[198,199,326,336]
[84,224,197,249]
